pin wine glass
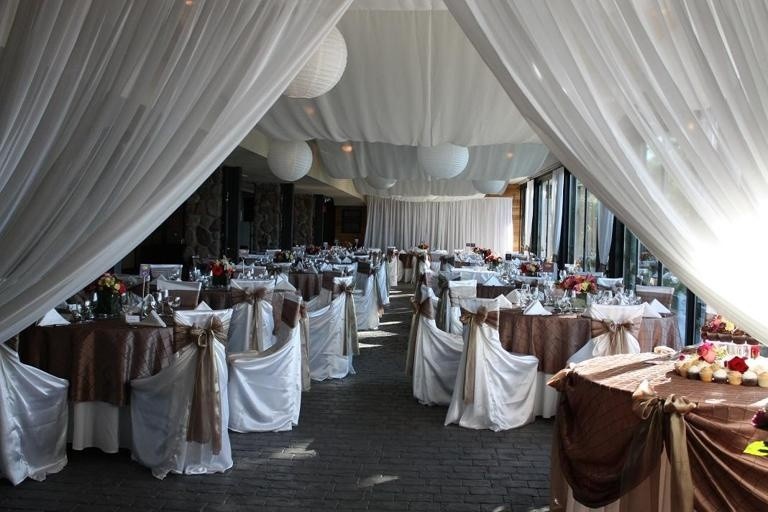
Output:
[521,281,634,314]
[76,289,181,321]
